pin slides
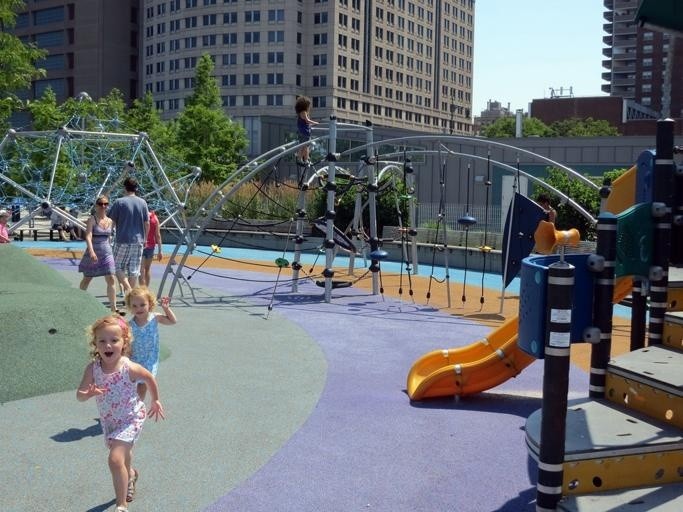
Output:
[407,163,636,400]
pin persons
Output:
[78,196,126,316]
[537,194,557,226]
[50,206,83,241]
[108,178,150,291]
[0,210,10,243]
[295,96,319,165]
[76,315,165,512]
[116,280,125,297]
[139,212,163,286]
[124,286,177,402]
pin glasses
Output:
[97,201,110,206]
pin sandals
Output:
[126,468,138,503]
[114,506,128,512]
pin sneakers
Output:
[116,292,124,297]
[297,157,312,165]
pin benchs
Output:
[16,227,62,241]
[393,227,502,257]
[157,216,295,240]
